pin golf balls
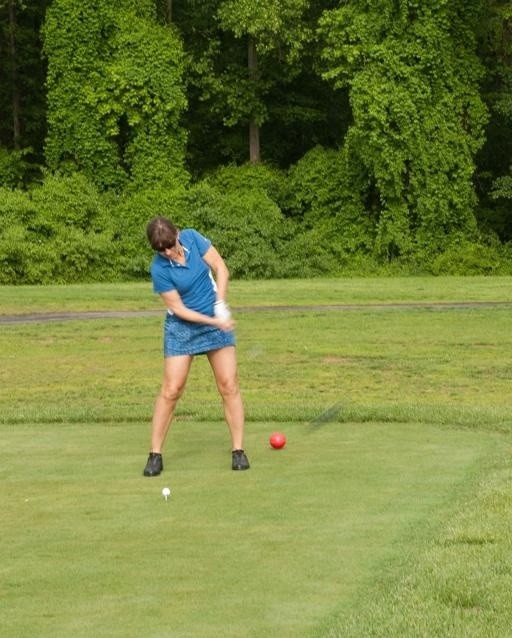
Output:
[269,433,287,448]
[162,487,170,495]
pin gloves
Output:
[213,301,232,321]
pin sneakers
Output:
[230,450,249,470]
[142,452,163,477]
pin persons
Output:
[141,217,250,477]
